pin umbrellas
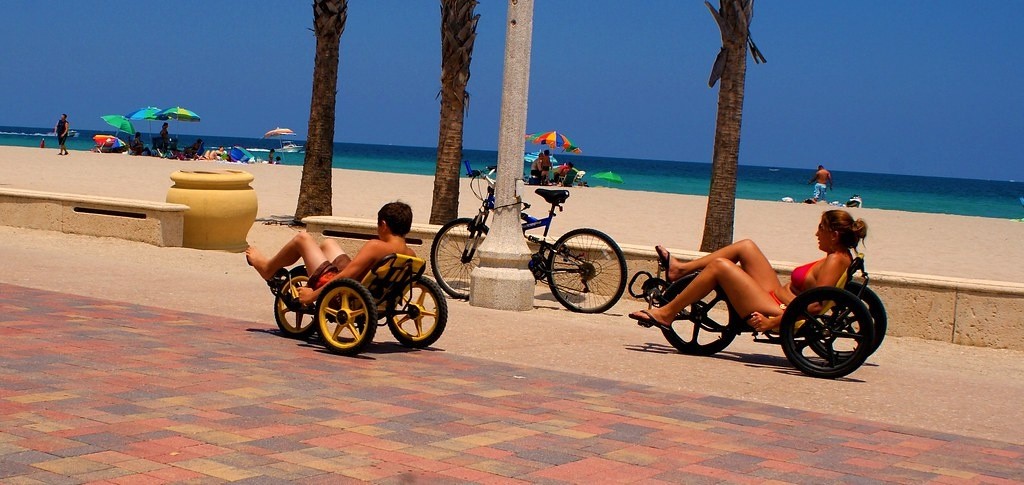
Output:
[122,105,162,146]
[153,105,201,138]
[525,130,582,170]
[591,170,624,187]
[524,148,557,165]
[264,126,296,164]
[552,164,586,179]
[98,114,136,137]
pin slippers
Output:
[655,246,672,285]
[629,310,670,333]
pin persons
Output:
[245,198,417,312]
[268,148,275,164]
[538,150,552,183]
[530,153,552,186]
[808,164,834,203]
[553,161,574,184]
[629,209,868,332]
[275,155,281,164]
[133,123,229,161]
[54,114,69,156]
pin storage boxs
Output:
[529,176,539,185]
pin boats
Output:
[245,148,270,152]
[67,129,77,136]
[275,140,304,153]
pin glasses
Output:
[819,223,836,232]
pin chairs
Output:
[94,137,115,154]
[557,170,590,187]
[128,136,140,157]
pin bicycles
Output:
[430,164,629,315]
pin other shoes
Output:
[58,153,62,155]
[64,152,68,155]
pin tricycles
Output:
[628,245,889,380]
[245,251,449,357]
